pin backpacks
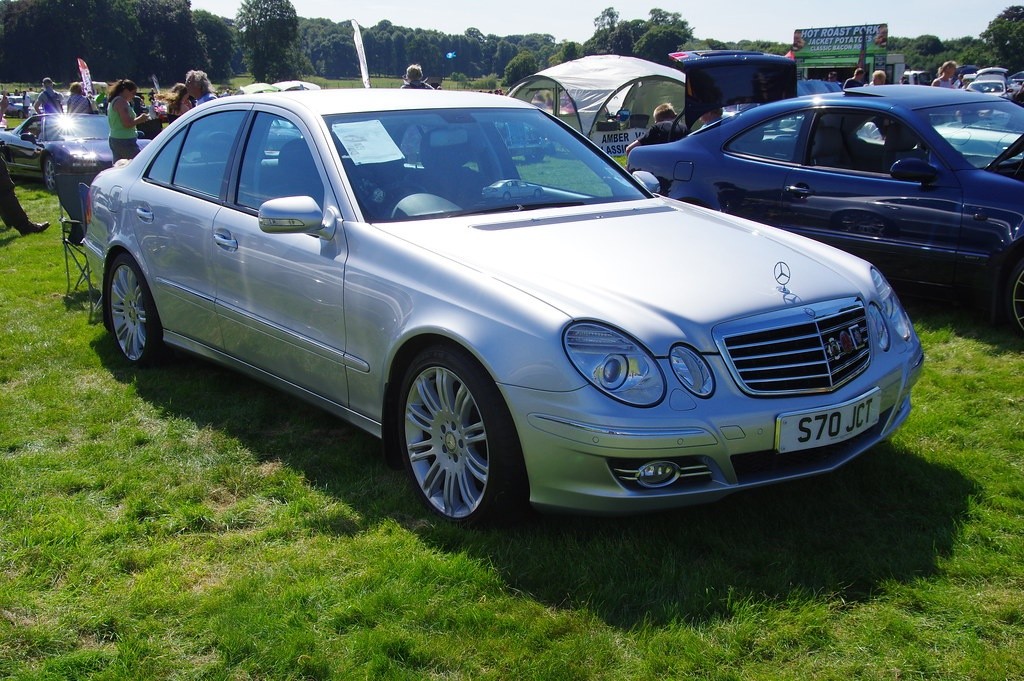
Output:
[44,91,63,113]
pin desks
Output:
[593,129,649,156]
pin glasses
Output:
[188,80,200,84]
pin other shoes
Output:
[21,222,50,236]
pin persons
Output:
[0,95,51,235]
[899,76,910,85]
[479,89,504,96]
[400,64,443,90]
[533,90,552,107]
[930,60,965,90]
[0,70,233,126]
[828,71,838,82]
[107,79,150,165]
[843,68,886,89]
[1017,81,1024,96]
[699,108,723,130]
[626,103,687,156]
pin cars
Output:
[903,67,1024,106]
[619,46,1024,338]
[0,112,158,193]
[76,87,929,526]
[0,95,44,118]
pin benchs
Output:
[176,130,278,211]
[741,124,857,166]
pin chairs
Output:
[883,124,917,164]
[808,128,827,165]
[51,162,103,314]
[276,138,327,212]
[419,129,490,206]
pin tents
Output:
[504,55,687,156]
[235,81,321,95]
